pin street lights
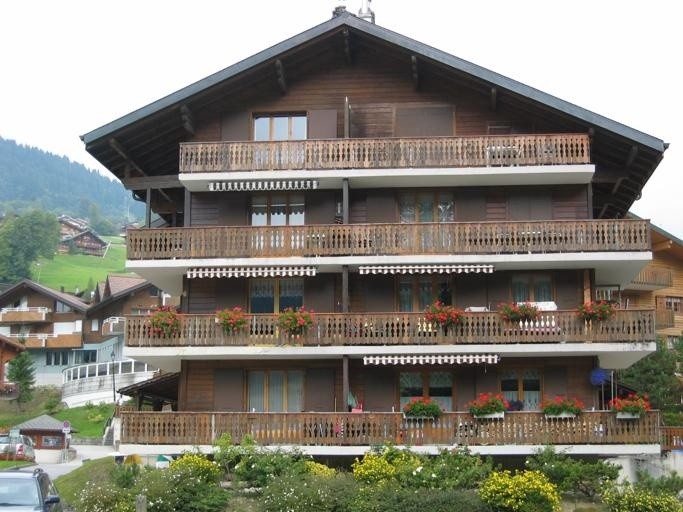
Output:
[111,351,115,401]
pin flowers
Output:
[403,392,652,415]
[419,299,468,327]
[575,299,622,321]
[496,299,542,323]
[214,304,254,336]
[275,304,318,342]
[145,305,182,337]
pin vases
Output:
[402,412,641,419]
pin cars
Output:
[0,464,63,512]
[0,434,35,462]
